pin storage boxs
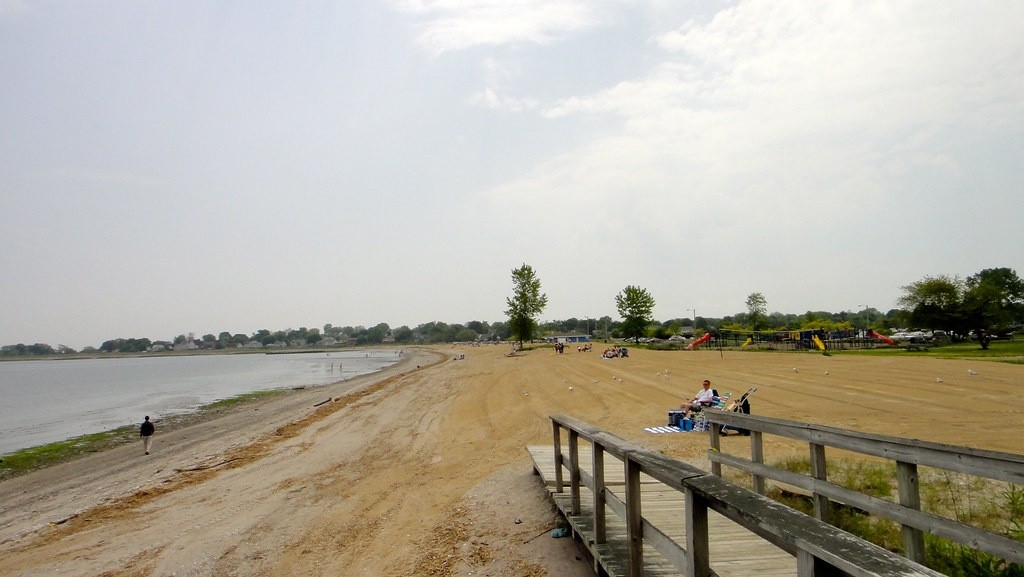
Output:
[680,419,693,431]
[667,411,685,426]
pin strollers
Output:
[718,385,757,436]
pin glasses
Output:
[703,384,708,386]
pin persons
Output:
[603,345,623,359]
[577,343,592,352]
[554,343,564,353]
[680,380,713,412]
[140,416,155,455]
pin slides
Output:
[872,331,896,345]
[812,335,825,350]
[742,338,752,348]
[690,333,711,347]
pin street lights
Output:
[858,303,870,331]
[687,309,698,350]
[593,318,598,339]
[584,315,589,341]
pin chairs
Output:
[693,390,731,426]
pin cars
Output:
[887,330,956,343]
